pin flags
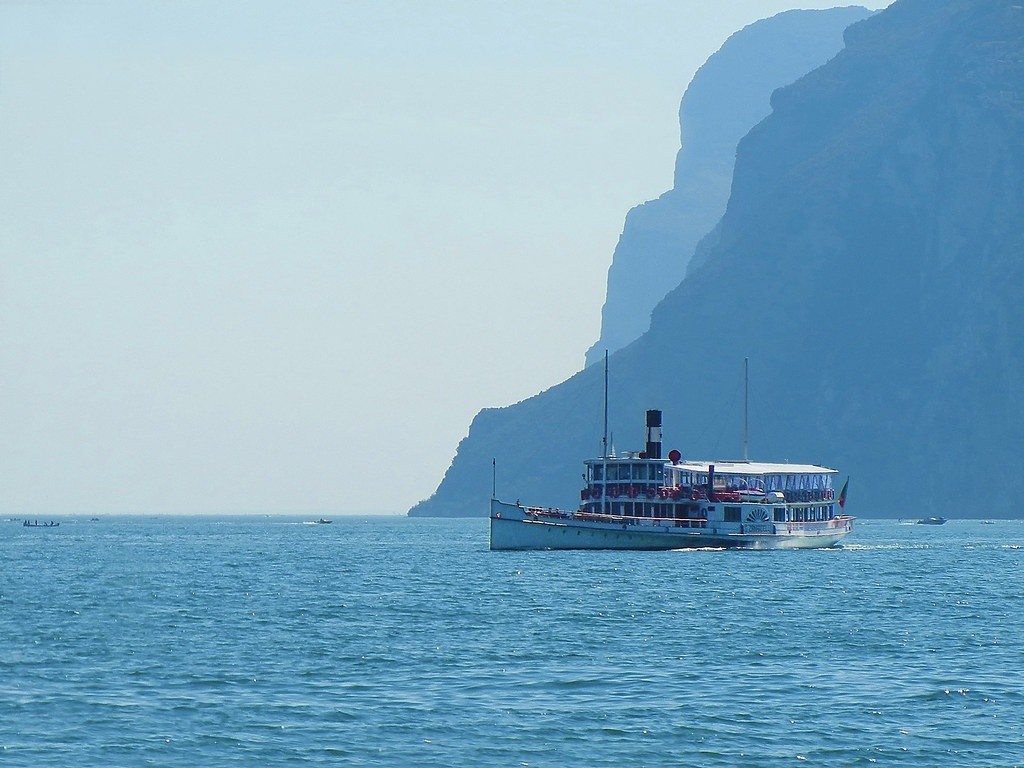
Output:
[838,480,849,507]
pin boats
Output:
[313,519,333,524]
[916,515,948,525]
[488,349,860,552]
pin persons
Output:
[526,505,562,519]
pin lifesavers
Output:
[689,489,701,501]
[582,490,590,499]
[646,489,655,498]
[659,484,682,500]
[607,486,620,498]
[592,489,602,499]
[821,488,833,501]
[627,487,638,498]
